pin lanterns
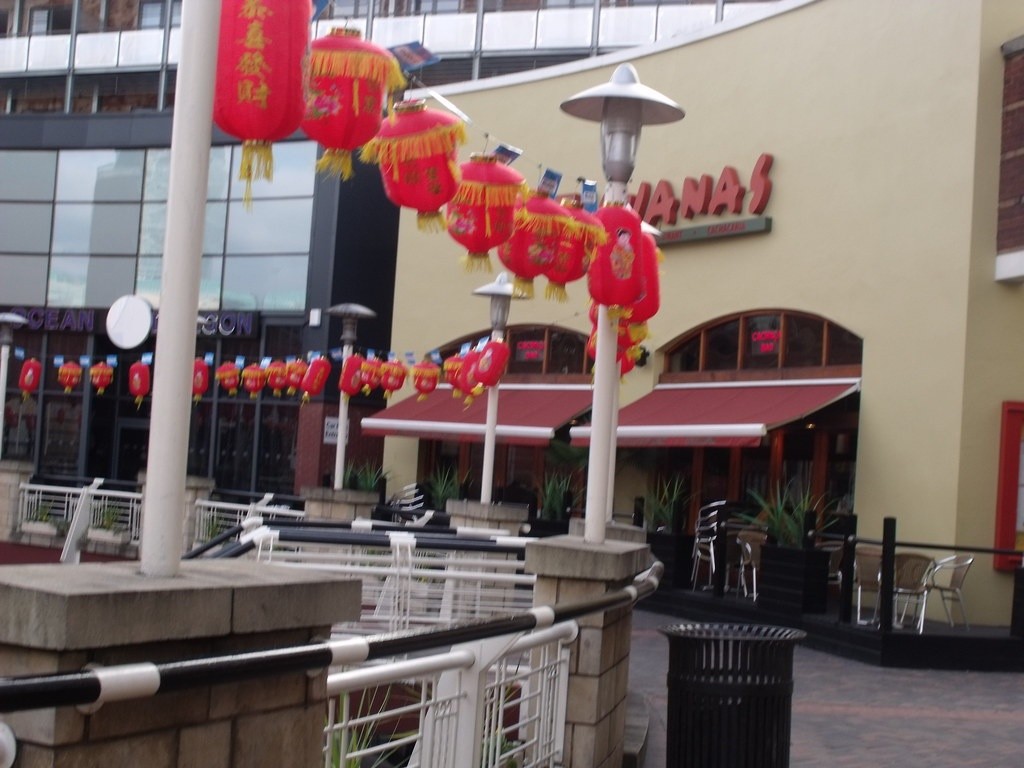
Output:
[19,356,151,410]
[191,338,511,412]
[215,0,664,377]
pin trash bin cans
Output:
[654,620,809,768]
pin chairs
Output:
[389,482,425,522]
[632,501,974,635]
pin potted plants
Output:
[21,497,64,536]
[421,465,473,526]
[646,471,702,599]
[192,511,229,556]
[731,478,831,614]
[88,498,132,544]
[533,460,586,537]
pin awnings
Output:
[572,379,862,447]
[361,381,592,446]
